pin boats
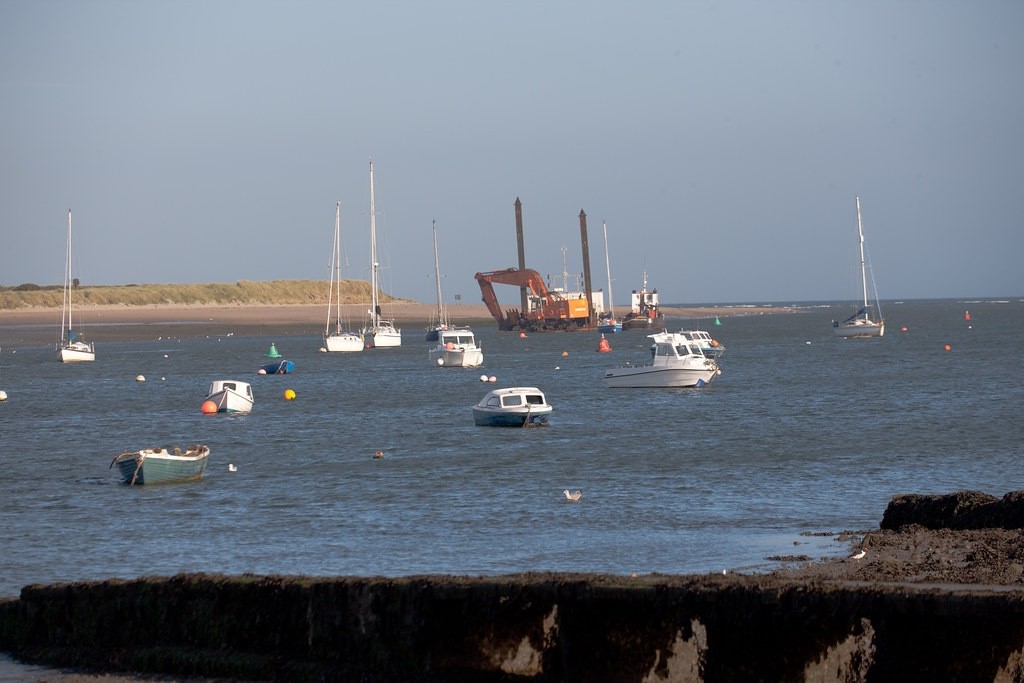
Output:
[604,341,721,388]
[114,444,211,484]
[622,271,666,330]
[260,361,295,374]
[428,304,484,368]
[204,379,255,413]
[472,387,553,427]
[650,327,727,360]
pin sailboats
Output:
[597,218,622,333]
[831,194,884,337]
[364,159,402,349]
[54,208,97,363]
[321,201,364,351]
[426,218,470,341]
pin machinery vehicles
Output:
[474,268,589,330]
